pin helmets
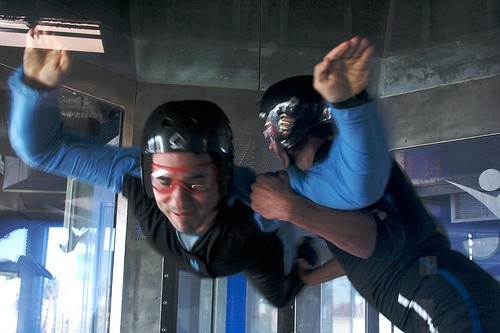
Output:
[258,75,336,153]
[137,100,234,168]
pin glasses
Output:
[149,160,220,195]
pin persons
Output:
[248,74,500,332]
[7,22,393,308]
